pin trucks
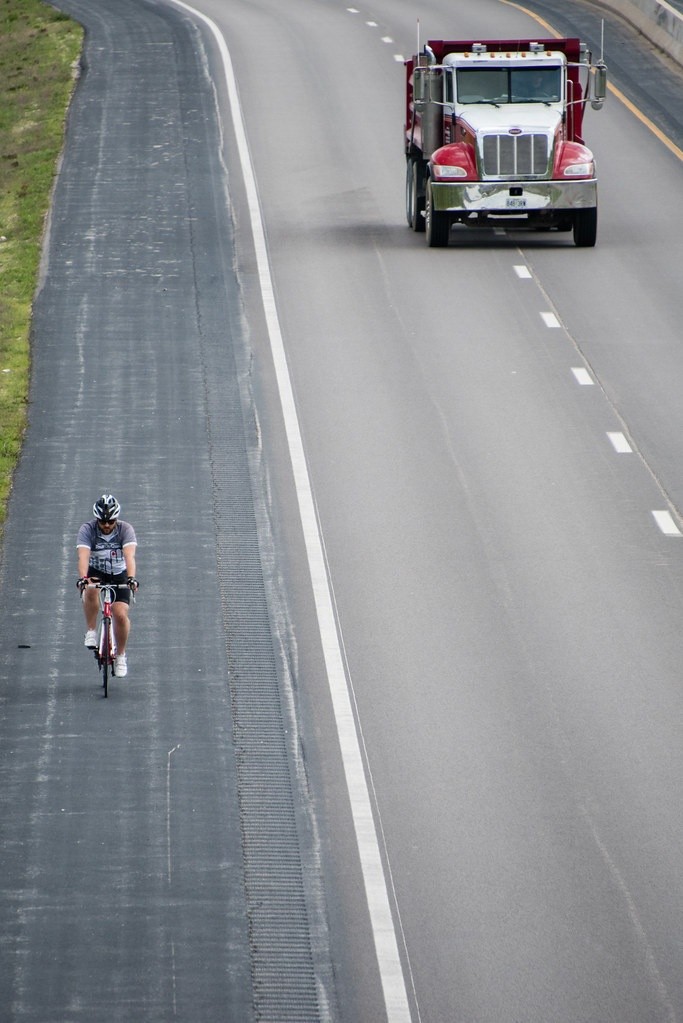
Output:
[402,17,608,249]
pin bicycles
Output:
[77,576,138,699]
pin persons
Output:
[76,494,138,676]
[523,72,544,98]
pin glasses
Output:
[98,519,117,525]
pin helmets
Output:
[93,495,120,520]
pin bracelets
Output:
[128,576,133,579]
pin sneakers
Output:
[114,655,127,678]
[84,629,98,647]
